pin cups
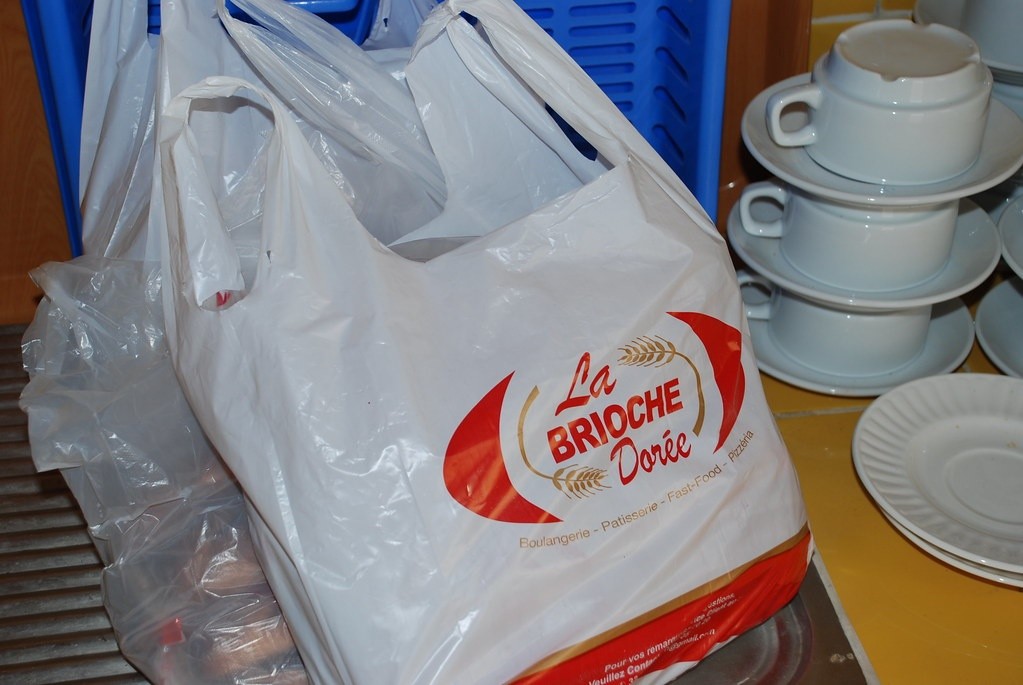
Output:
[739,181,959,292]
[732,273,930,378]
[959,0,1023,65]
[766,19,994,186]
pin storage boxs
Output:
[16,0,729,258]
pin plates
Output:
[740,71,1023,207]
[913,2,1023,84]
[975,278,1023,378]
[852,372,1023,589]
[737,284,975,396]
[727,182,1004,312]
[993,188,1022,280]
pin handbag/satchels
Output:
[20,1,814,685]
